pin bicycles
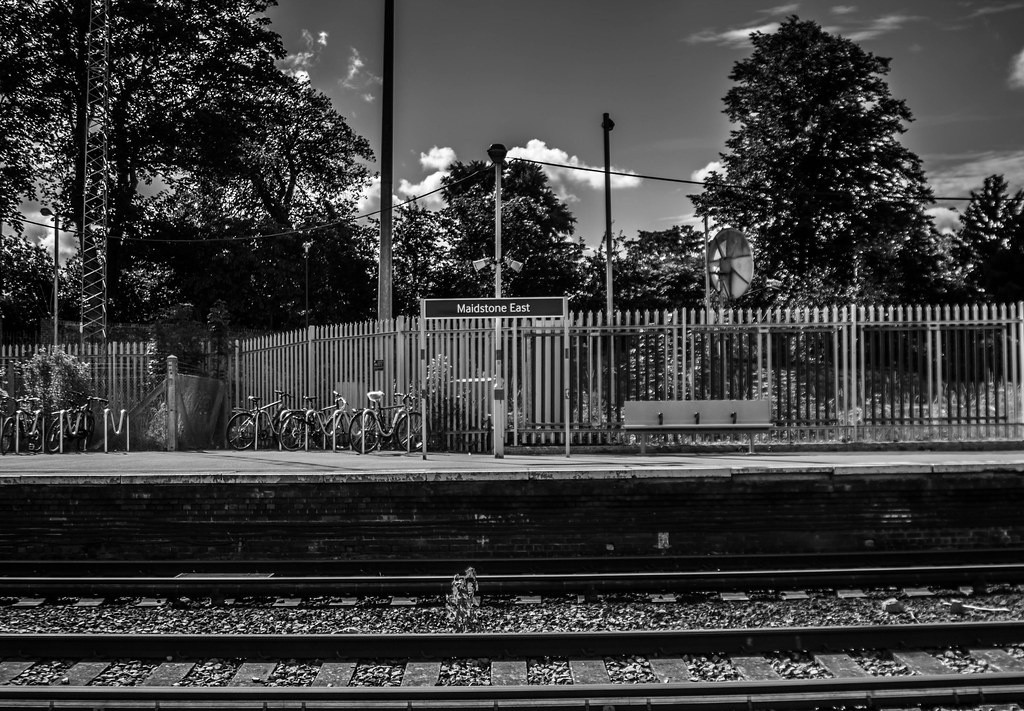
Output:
[0,397,109,455]
[225,389,429,453]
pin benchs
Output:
[623,401,775,456]
